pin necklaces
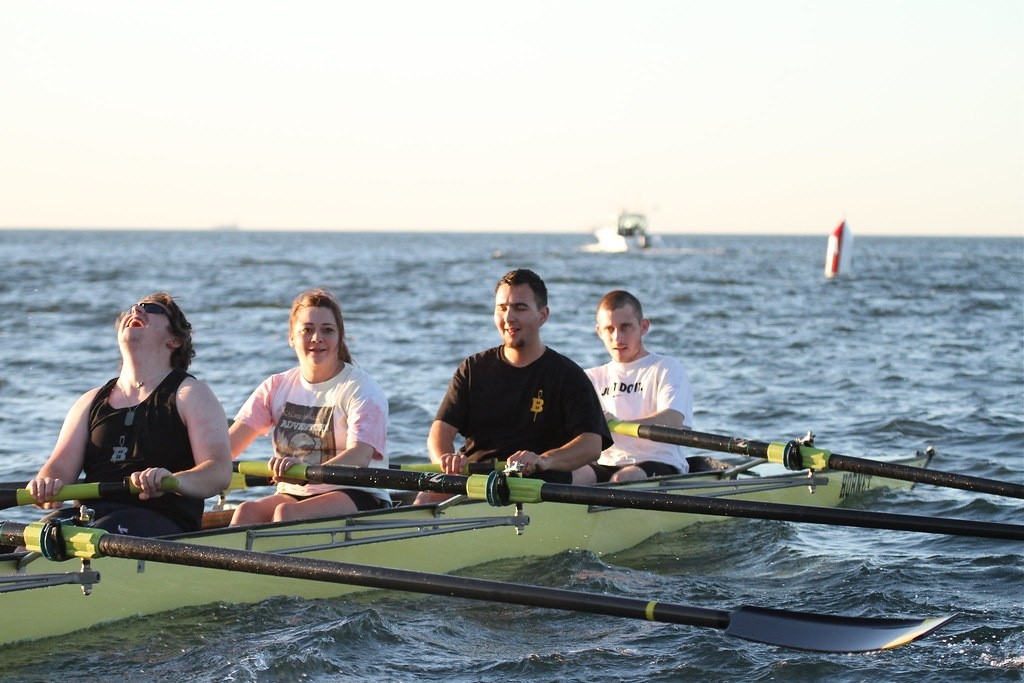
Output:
[118,374,166,426]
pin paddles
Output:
[610,422,1024,500]
[233,460,1024,544]
[0,519,960,653]
[0,475,178,511]
[388,460,508,475]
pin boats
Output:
[0,446,936,646]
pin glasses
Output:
[124,302,175,334]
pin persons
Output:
[572,291,694,486]
[230,289,393,527]
[413,269,615,506]
[12,291,232,555]
